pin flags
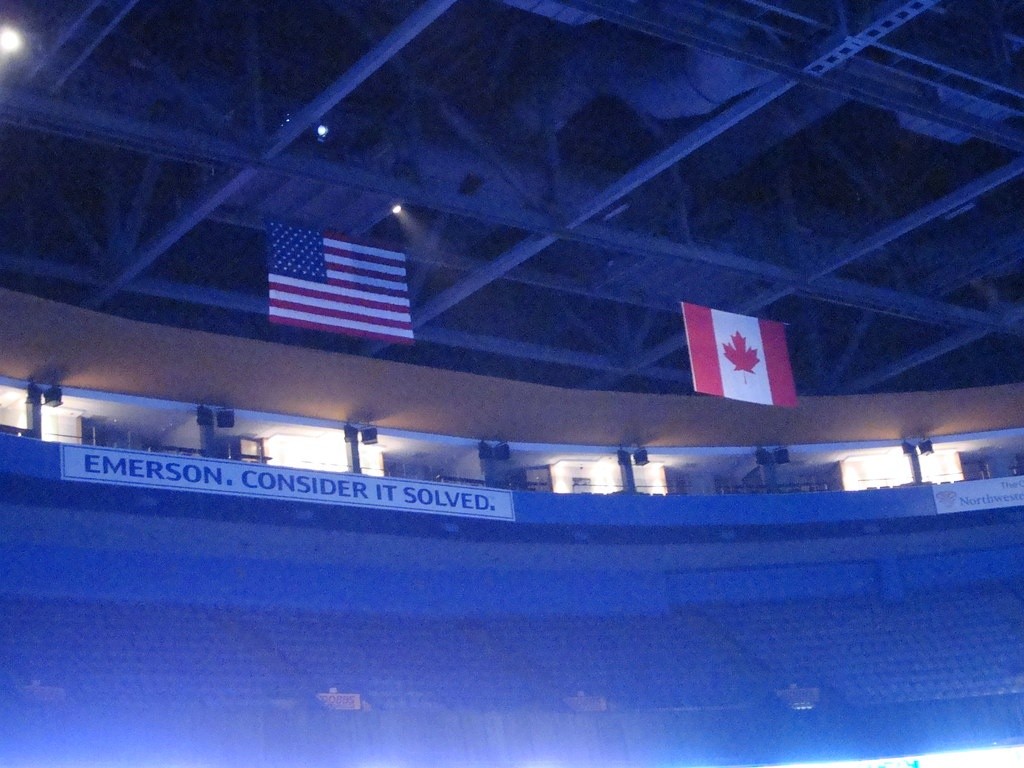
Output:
[267,219,414,343]
[682,302,799,407]
